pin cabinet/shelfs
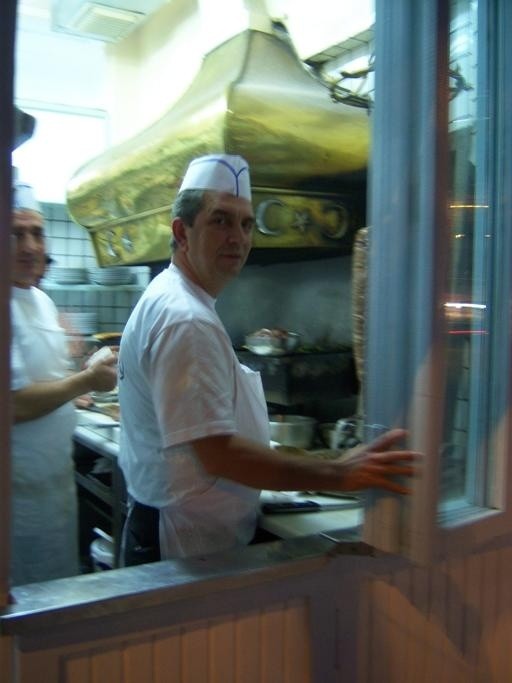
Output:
[73,401,363,538]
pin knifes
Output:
[262,501,366,515]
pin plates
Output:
[48,267,133,287]
[60,312,97,337]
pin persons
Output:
[12,182,119,586]
[117,154,425,568]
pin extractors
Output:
[68,29,377,268]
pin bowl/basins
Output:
[243,334,300,357]
[267,415,357,448]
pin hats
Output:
[177,155,252,203]
[11,183,42,213]
